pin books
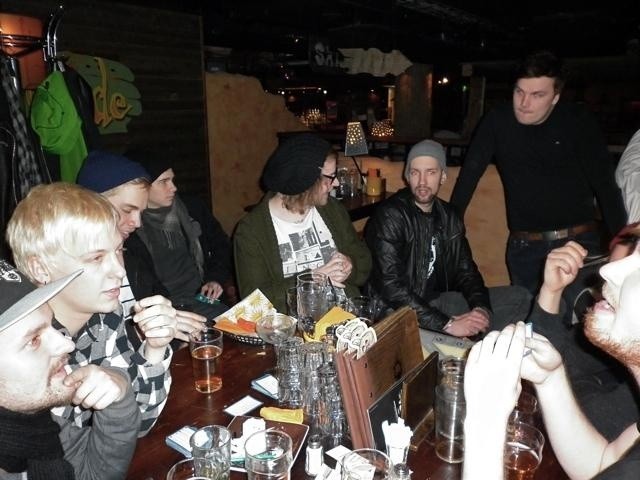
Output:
[333,305,439,472]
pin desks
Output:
[363,134,422,165]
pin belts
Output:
[511,223,593,241]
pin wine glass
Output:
[255,314,296,377]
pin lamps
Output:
[343,120,387,198]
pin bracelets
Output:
[442,317,455,332]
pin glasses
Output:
[322,173,337,184]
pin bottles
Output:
[394,464,410,480]
[305,433,324,476]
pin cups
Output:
[434,384,466,464]
[385,446,408,467]
[366,177,381,196]
[287,271,373,335]
[273,336,345,436]
[189,327,224,394]
[346,173,357,197]
[338,168,348,194]
[435,357,466,440]
[502,422,545,479]
[341,447,393,480]
[245,429,293,480]
[167,458,220,479]
[368,168,378,178]
[368,196,380,203]
[190,425,231,479]
[509,393,538,427]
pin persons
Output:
[232,133,375,315]
[0,180,179,442]
[528,227,640,445]
[613,122,640,230]
[458,239,640,479]
[361,138,531,350]
[0,261,141,479]
[447,55,628,293]
[128,154,231,330]
[75,154,212,343]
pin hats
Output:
[262,135,330,195]
[124,150,172,186]
[76,150,146,193]
[407,140,446,170]
[0,259,84,332]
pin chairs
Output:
[433,70,487,167]
